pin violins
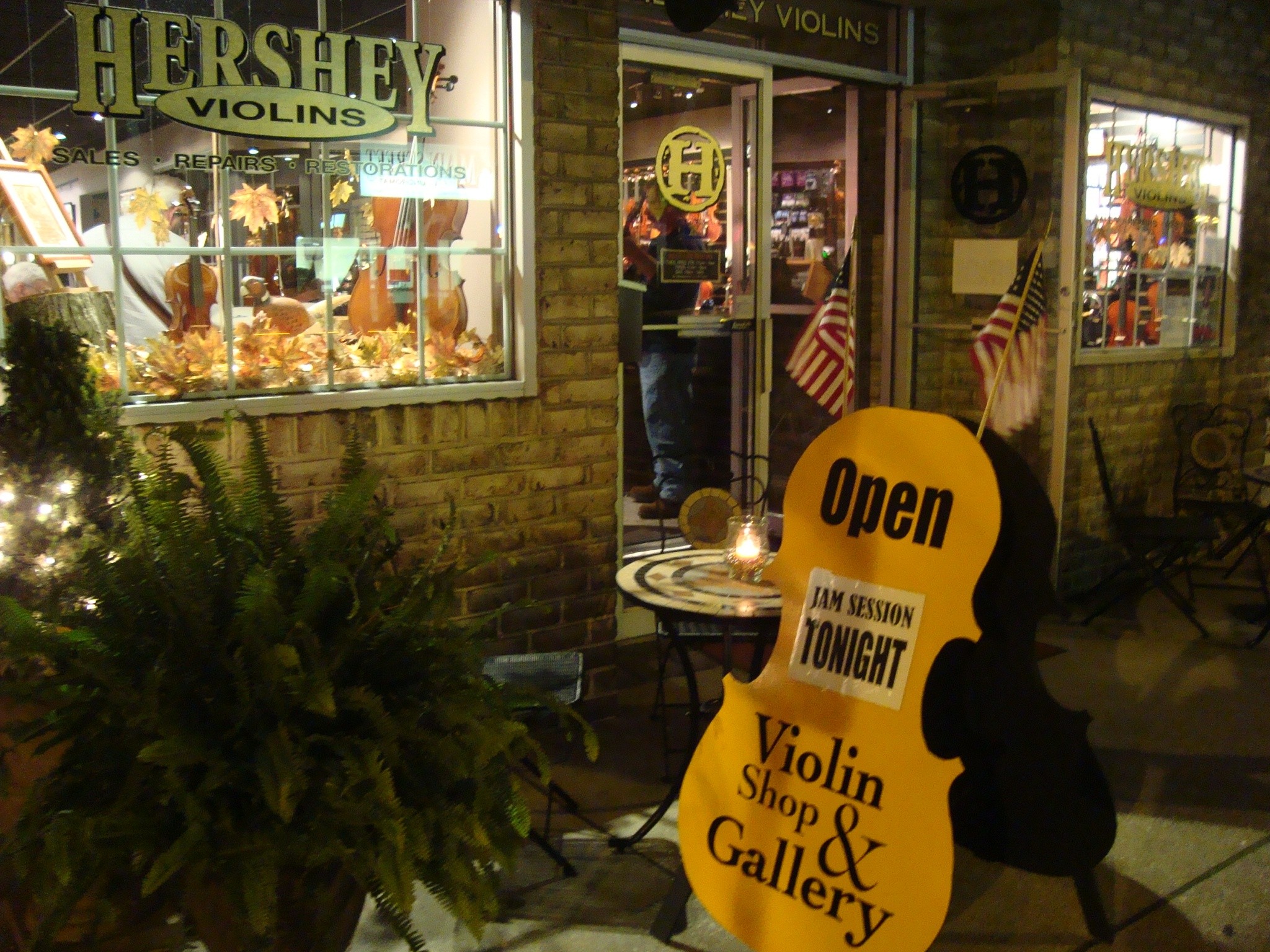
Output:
[1147,280,1162,342]
[348,63,469,353]
[1144,145,1189,269]
[159,185,218,346]
[620,160,732,314]
[1106,255,1144,347]
[1117,127,1155,255]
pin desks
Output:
[605,548,783,851]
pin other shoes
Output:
[630,484,660,502]
[638,497,682,519]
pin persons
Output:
[623,177,814,519]
[3,262,51,302]
[68,175,205,347]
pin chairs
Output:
[1079,404,1270,649]
[647,453,775,776]
[478,649,583,877]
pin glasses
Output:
[25,284,51,295]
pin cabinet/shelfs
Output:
[622,155,836,274]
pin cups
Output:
[725,514,770,584]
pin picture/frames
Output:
[0,160,95,274]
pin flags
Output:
[970,240,1051,441]
[787,235,855,416]
